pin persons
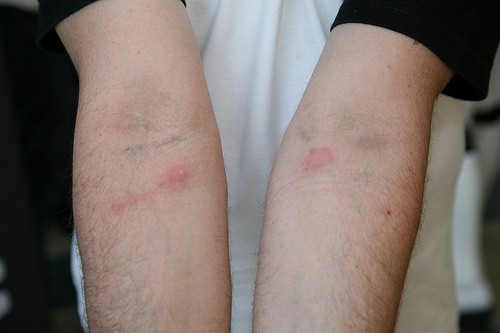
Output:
[36,0,500,333]
